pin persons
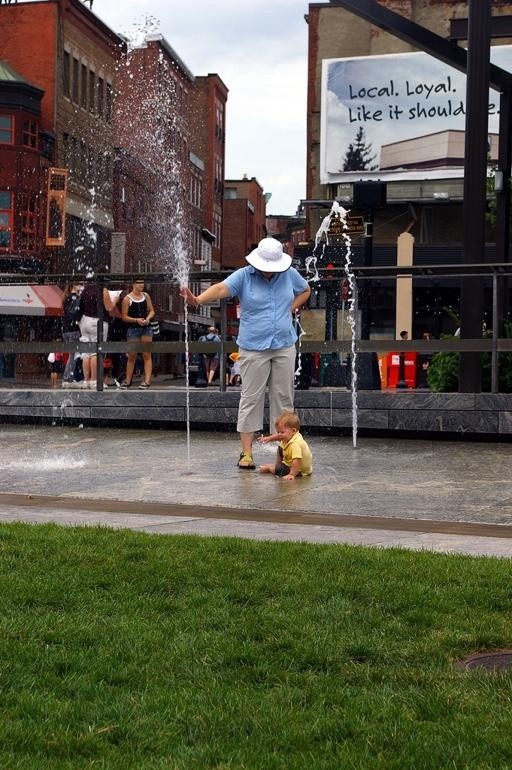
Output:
[228,352,242,385]
[49,271,155,389]
[179,237,312,470]
[198,326,221,384]
[257,410,313,481]
[399,331,409,340]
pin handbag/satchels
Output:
[48,352,56,363]
[149,319,160,334]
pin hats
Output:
[244,238,292,273]
[208,327,218,334]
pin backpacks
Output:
[205,336,217,356]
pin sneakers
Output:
[237,451,255,469]
[62,379,132,389]
[138,383,150,389]
